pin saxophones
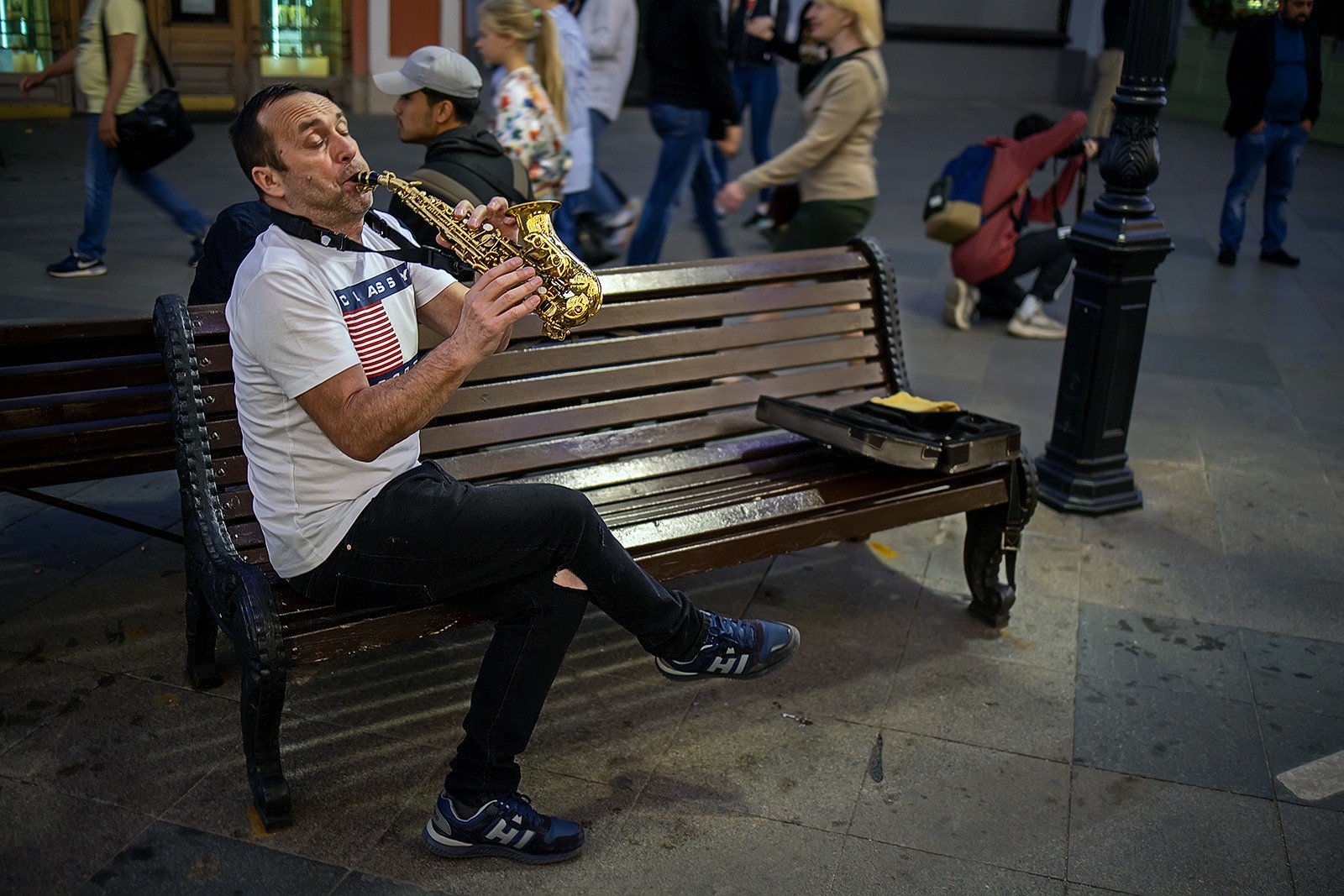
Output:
[349,168,606,344]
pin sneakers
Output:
[188,225,213,268]
[942,277,978,331]
[1007,303,1068,341]
[655,610,801,684]
[422,789,588,865]
[46,246,108,278]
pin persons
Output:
[940,109,1098,341]
[474,0,831,290]
[1101,0,1324,271]
[224,84,799,866]
[714,0,888,401]
[371,45,535,250]
[19,0,218,278]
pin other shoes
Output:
[692,209,730,228]
[1217,242,1237,267]
[1259,248,1300,267]
[599,199,642,232]
[742,206,775,231]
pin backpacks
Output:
[923,144,1032,245]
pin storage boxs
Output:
[754,394,1021,475]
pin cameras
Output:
[1056,135,1103,159]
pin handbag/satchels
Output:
[569,211,621,268]
[109,89,195,177]
[761,182,802,244]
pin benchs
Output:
[0,318,183,544]
[151,235,1039,835]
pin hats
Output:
[372,45,484,99]
